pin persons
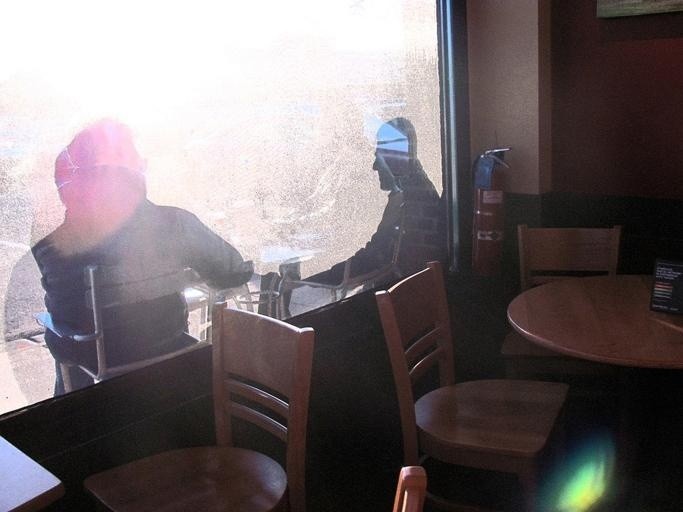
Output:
[301,117,442,282]
[32,120,253,398]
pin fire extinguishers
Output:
[470,147,511,277]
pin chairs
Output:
[376,259,571,511]
[501,223,623,386]
[79,298,316,511]
[35,219,452,386]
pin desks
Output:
[507,267,680,373]
